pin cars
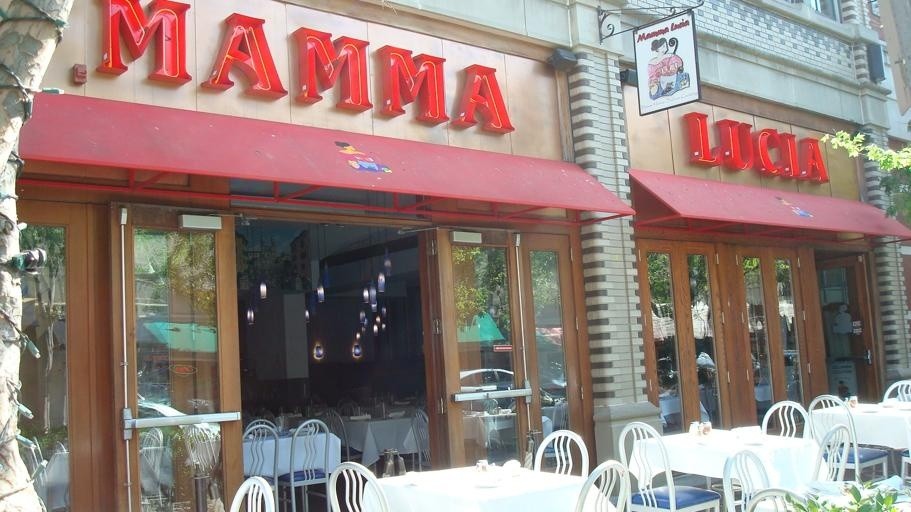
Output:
[458,367,515,394]
[782,349,798,375]
[537,378,565,397]
[137,398,222,484]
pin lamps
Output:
[545,48,578,69]
[621,68,639,87]
[243,191,394,337]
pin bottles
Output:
[687,420,716,444]
[474,457,498,488]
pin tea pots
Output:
[844,395,859,410]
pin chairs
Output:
[229,390,626,510]
[618,381,911,510]
[16,418,222,511]
